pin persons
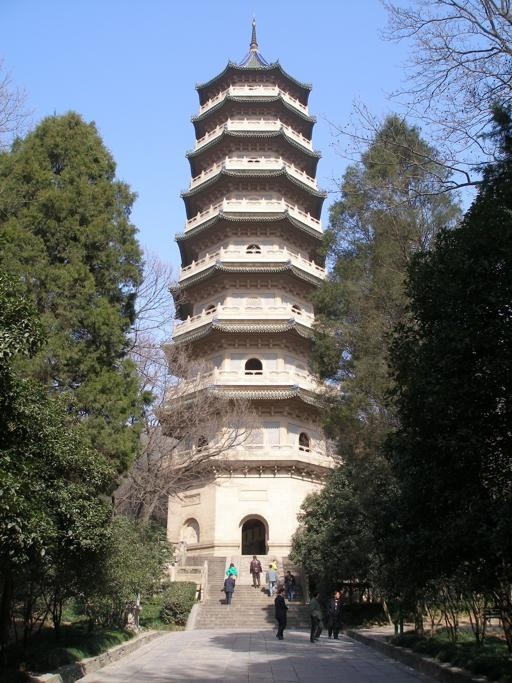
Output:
[308,590,324,643]
[249,554,263,588]
[273,587,289,640]
[265,563,277,596]
[271,558,280,574]
[325,590,343,640]
[283,569,296,601]
[222,573,235,605]
[225,562,239,586]
